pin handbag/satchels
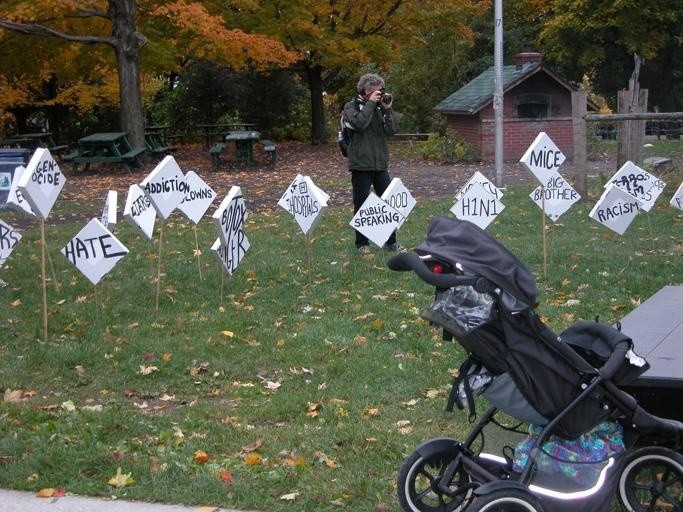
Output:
[336,127,350,157]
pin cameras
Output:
[378,88,392,105]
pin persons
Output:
[341,73,406,256]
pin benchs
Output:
[194,123,256,151]
[611,285,683,460]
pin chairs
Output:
[66,143,94,170]
[0,131,69,171]
[144,125,186,160]
[121,142,149,172]
[208,141,228,170]
[260,138,278,171]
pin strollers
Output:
[385,214,682,511]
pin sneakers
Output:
[357,243,374,259]
[381,242,407,255]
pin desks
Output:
[72,132,141,174]
[223,130,264,173]
